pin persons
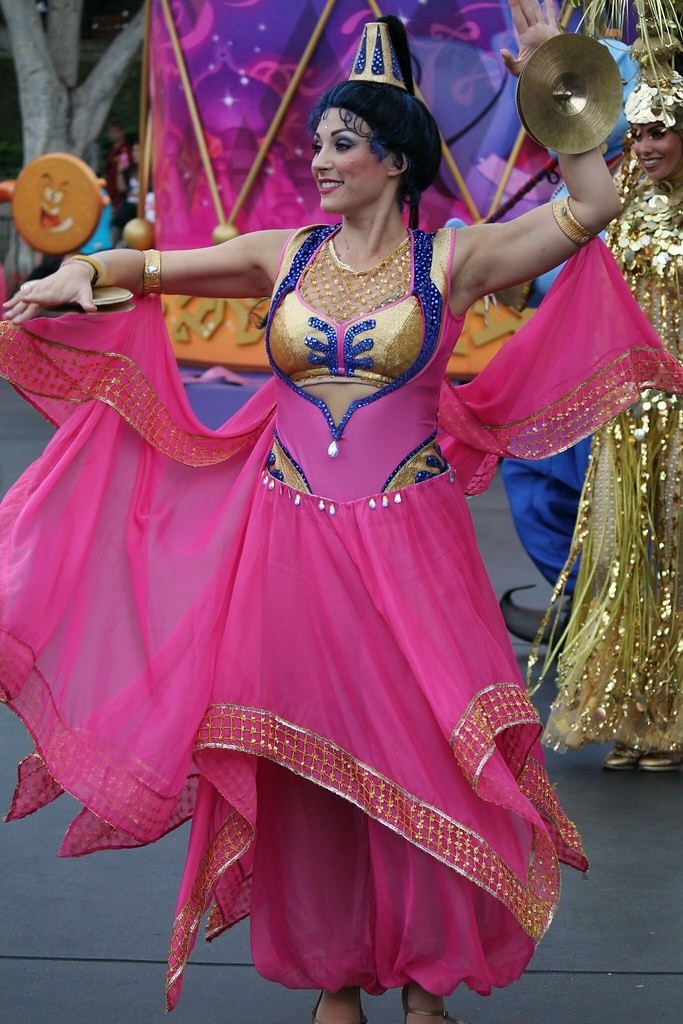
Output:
[3,1,623,1024]
[115,138,140,235]
[107,122,131,215]
[541,0,682,768]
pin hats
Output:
[565,0,683,124]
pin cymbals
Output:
[37,285,137,313]
[514,31,626,157]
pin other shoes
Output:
[599,740,683,771]
[402,985,469,1024]
[311,989,367,1024]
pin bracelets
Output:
[61,255,106,286]
